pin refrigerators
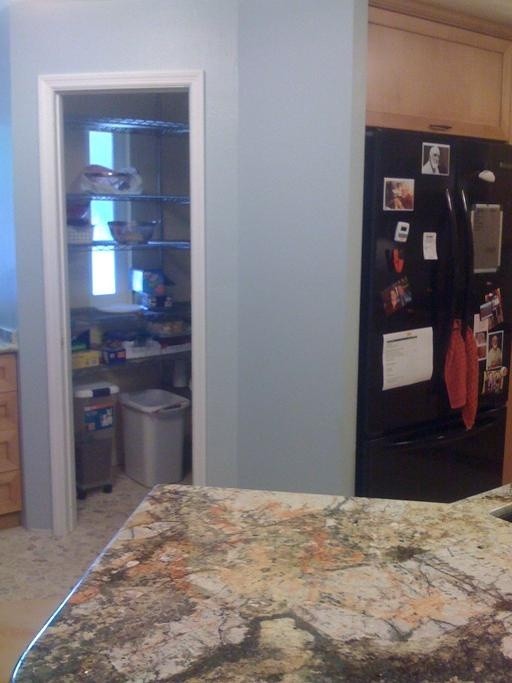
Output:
[351,122,511,502]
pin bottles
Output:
[164,297,173,309]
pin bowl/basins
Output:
[84,171,137,195]
[109,219,158,244]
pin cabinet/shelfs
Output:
[63,114,190,372]
[365,1,512,141]
[0,342,26,530]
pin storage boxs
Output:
[132,267,165,295]
[71,376,120,439]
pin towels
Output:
[445,318,479,428]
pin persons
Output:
[390,282,411,309]
[394,182,409,208]
[488,334,502,365]
[423,145,452,175]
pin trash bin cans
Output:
[118,388,191,488]
